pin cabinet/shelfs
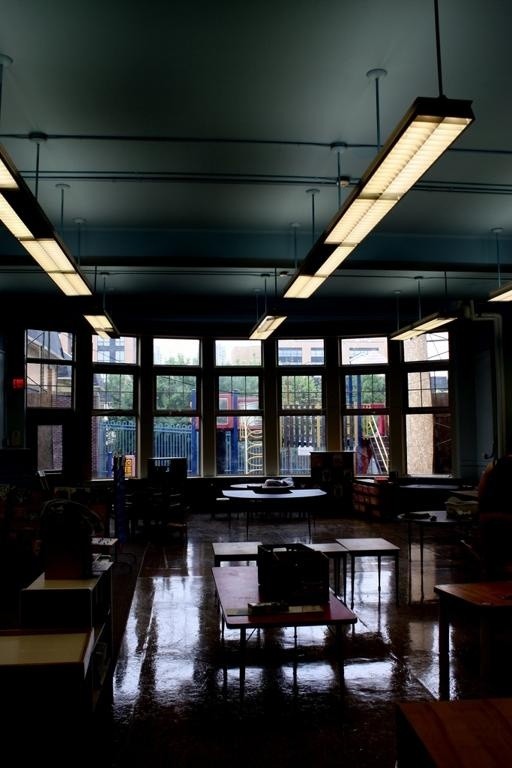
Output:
[146,456,188,487]
[307,449,357,512]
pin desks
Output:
[1,474,511,766]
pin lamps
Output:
[0,130,124,345]
[244,1,512,342]
